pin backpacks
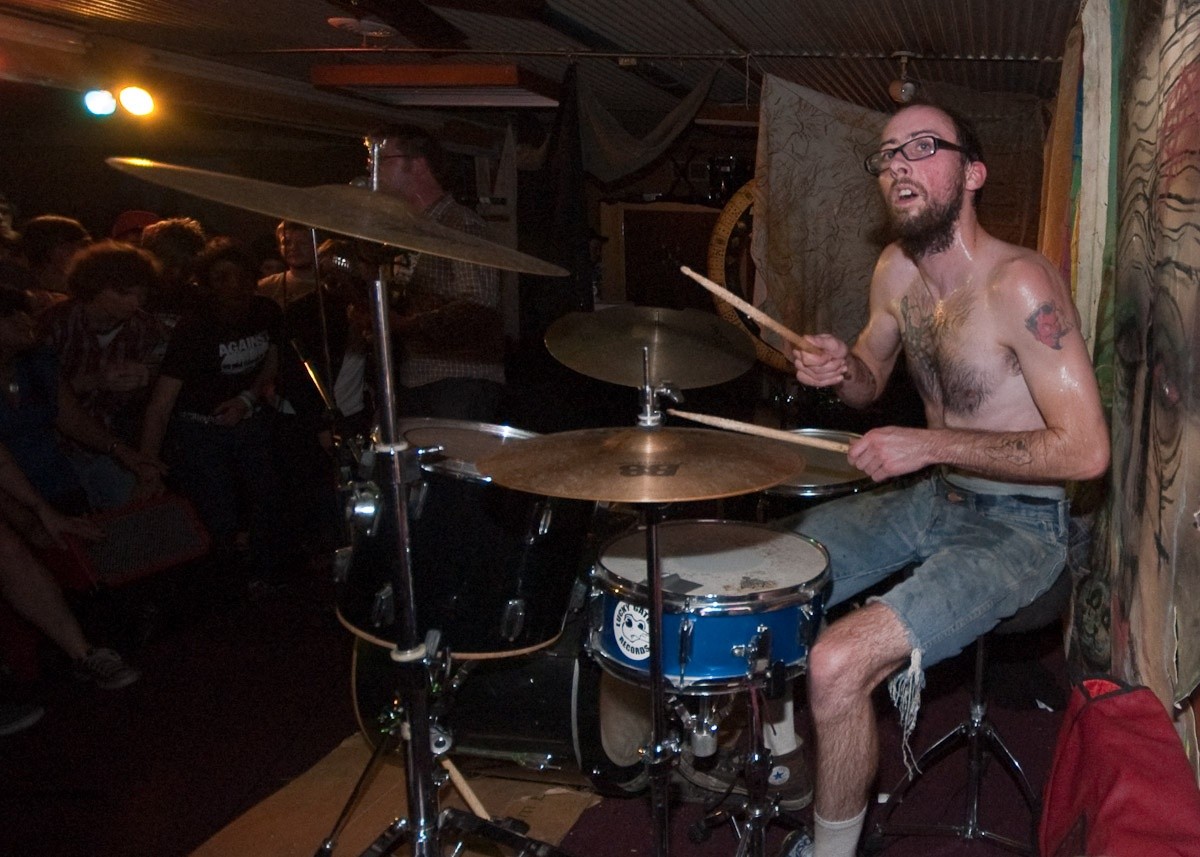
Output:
[1039,676,1200,857]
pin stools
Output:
[858,551,1076,857]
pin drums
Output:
[583,516,835,697]
[331,419,878,793]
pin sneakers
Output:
[69,647,139,688]
[665,730,813,810]
[0,705,44,736]
[778,830,814,857]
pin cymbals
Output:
[472,425,806,502]
[100,155,574,279]
[543,305,758,391]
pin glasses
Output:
[864,136,974,177]
[380,154,420,166]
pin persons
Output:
[674,101,1110,857]
[0,122,524,743]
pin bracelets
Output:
[237,392,257,409]
[100,437,119,453]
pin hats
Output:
[112,210,159,238]
